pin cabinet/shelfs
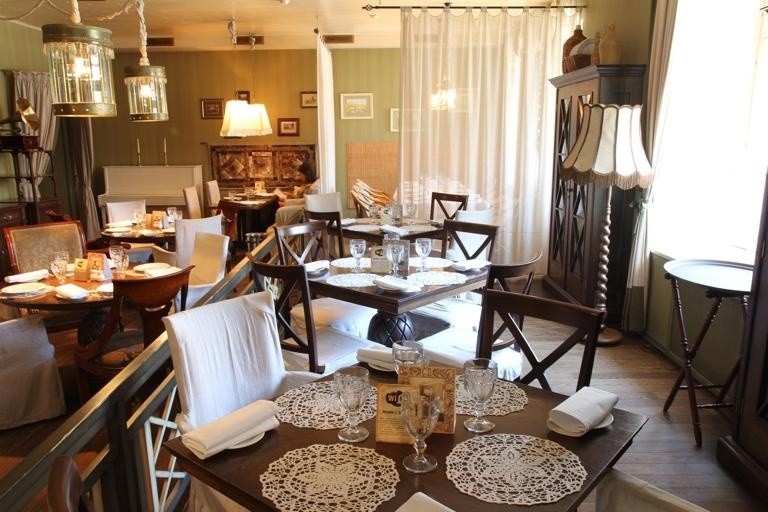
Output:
[538,60,649,336]
[709,161,766,508]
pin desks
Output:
[658,251,756,451]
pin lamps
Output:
[122,0,174,124]
[217,17,263,140]
[554,99,657,348]
[41,0,123,119]
[244,33,273,137]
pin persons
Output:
[277,164,316,206]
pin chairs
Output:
[1,180,304,510]
[243,179,500,374]
[163,291,324,430]
[417,252,544,381]
[595,468,705,510]
[475,289,606,393]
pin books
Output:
[273,188,287,201]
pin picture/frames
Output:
[340,91,374,121]
[234,89,252,105]
[277,117,300,137]
[200,97,224,119]
[390,108,422,132]
[299,90,317,109]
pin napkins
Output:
[182,398,284,461]
[546,386,620,438]
[396,492,451,510]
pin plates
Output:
[103,207,185,237]
[376,285,405,292]
[1,244,173,300]
[226,431,265,450]
[593,412,614,429]
[367,362,392,372]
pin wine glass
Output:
[463,357,498,434]
[335,366,370,443]
[350,231,432,277]
[401,388,441,474]
[368,203,418,228]
[226,187,276,206]
[392,340,424,377]
